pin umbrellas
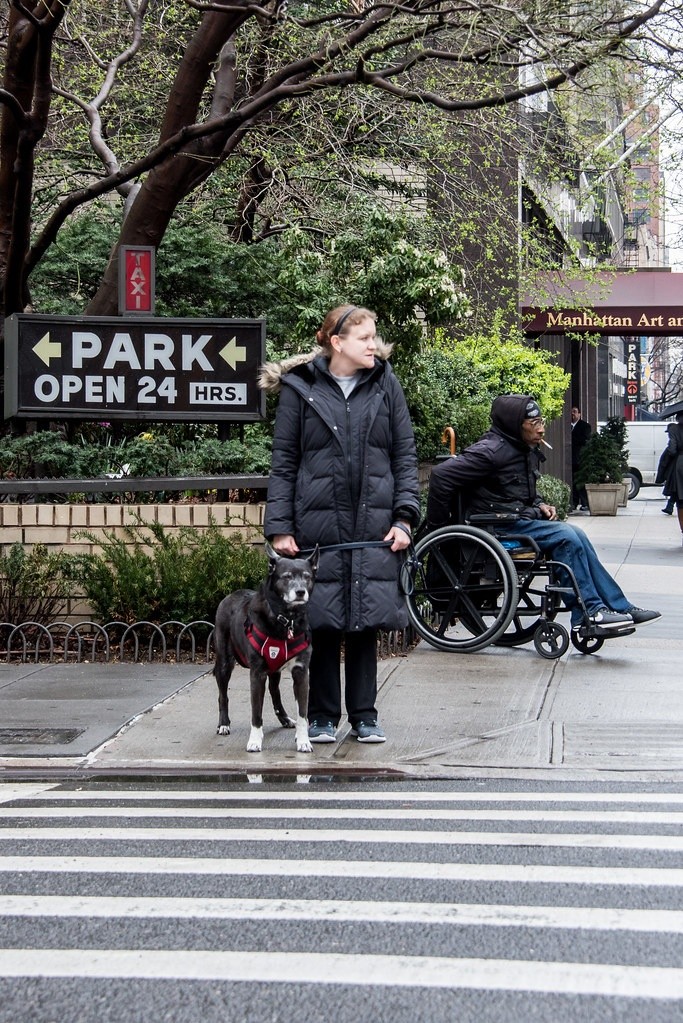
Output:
[657,400,683,418]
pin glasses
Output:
[528,417,546,429]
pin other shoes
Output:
[580,506,588,510]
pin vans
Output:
[596,420,679,500]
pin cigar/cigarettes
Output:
[539,439,553,450]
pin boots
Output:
[662,499,673,515]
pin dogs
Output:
[207,533,322,756]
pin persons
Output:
[661,413,683,532]
[423,392,664,633]
[260,302,423,743]
[570,406,593,512]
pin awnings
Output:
[523,272,683,336]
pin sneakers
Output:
[573,609,632,632]
[308,720,337,741]
[618,607,662,632]
[350,720,387,742]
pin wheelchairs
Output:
[399,454,637,659]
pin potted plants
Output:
[574,430,623,516]
[599,414,632,508]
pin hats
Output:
[665,423,675,432]
[523,400,542,419]
